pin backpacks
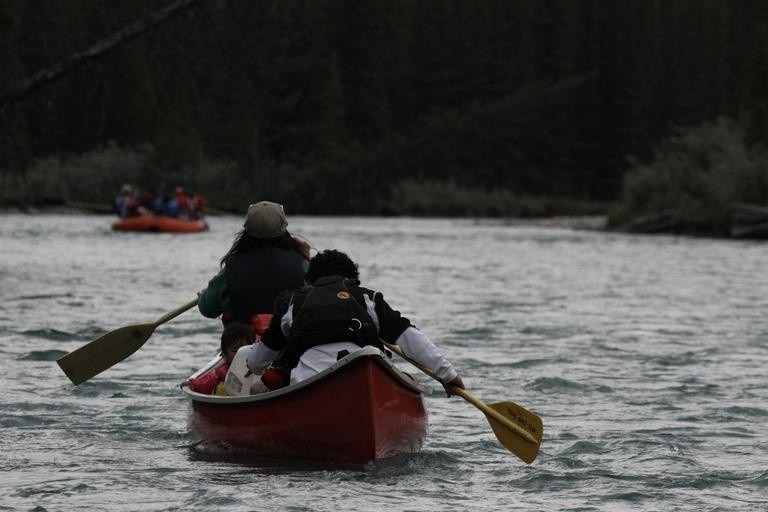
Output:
[288,275,375,347]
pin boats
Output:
[112,215,209,233]
[179,344,428,471]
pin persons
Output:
[180,321,282,395]
[242,248,466,400]
[196,200,311,328]
[114,182,207,223]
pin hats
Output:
[246,201,288,238]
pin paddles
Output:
[377,338,543,465]
[57,298,199,384]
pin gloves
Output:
[443,375,465,399]
